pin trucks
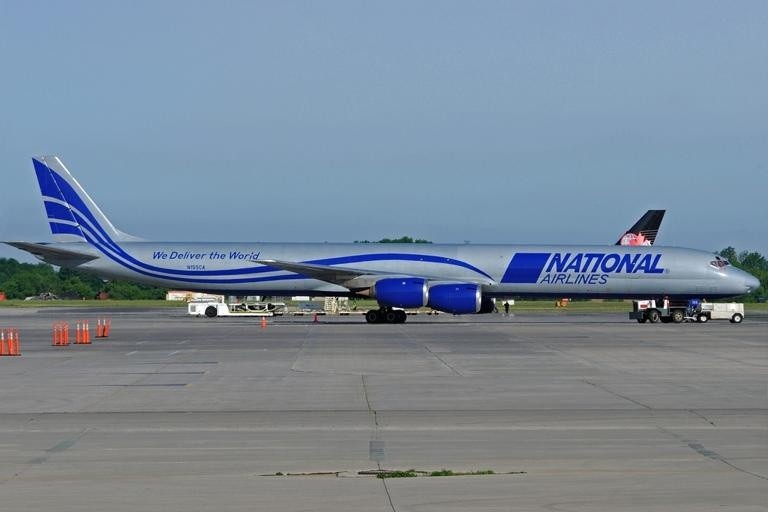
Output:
[629,298,693,324]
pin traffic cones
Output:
[259,315,267,327]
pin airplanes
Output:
[0,154,760,323]
